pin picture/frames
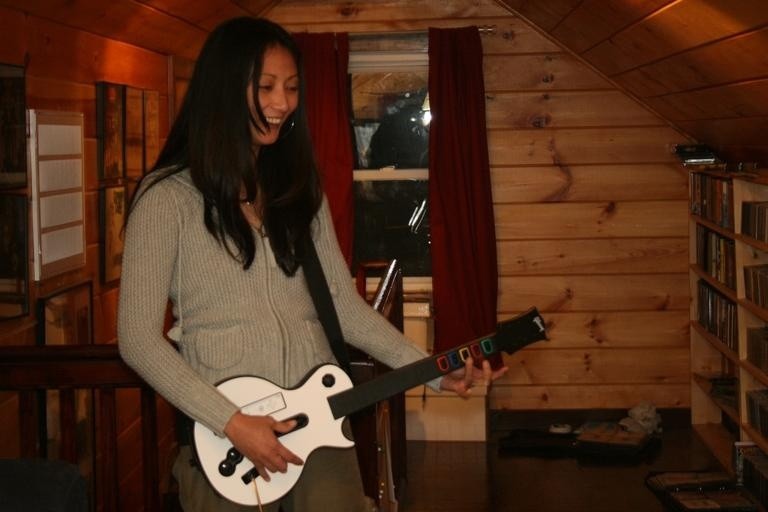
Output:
[95,79,127,182]
[98,184,131,287]
[124,83,146,181]
[37,279,99,490]
[144,89,163,173]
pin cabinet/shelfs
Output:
[730,170,768,509]
[685,169,740,479]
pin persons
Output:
[367,75,430,221]
[118,16,510,511]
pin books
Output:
[697,278,737,352]
[691,172,727,228]
[741,201,768,241]
[740,261,768,308]
[696,222,736,292]
[695,371,738,408]
[734,441,768,512]
[747,327,768,375]
[746,388,768,441]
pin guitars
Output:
[193,305,544,506]
